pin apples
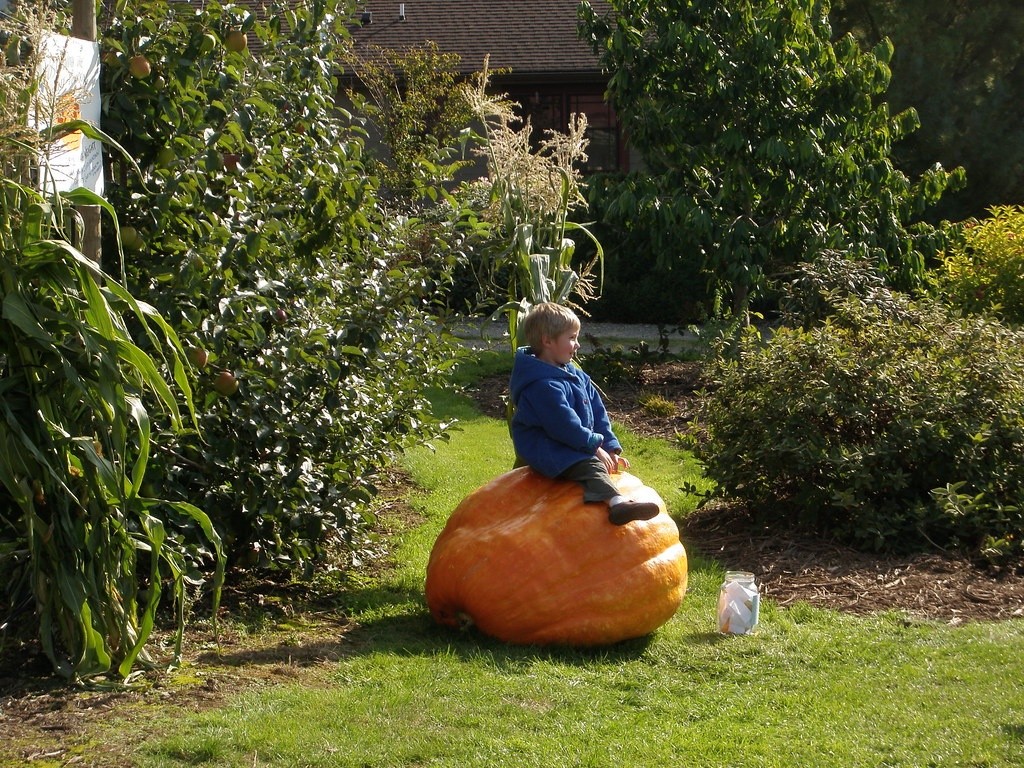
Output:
[104,31,289,396]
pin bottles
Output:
[718,571,760,634]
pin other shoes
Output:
[607,499,661,526]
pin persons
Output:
[508,301,662,527]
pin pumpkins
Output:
[423,466,689,646]
